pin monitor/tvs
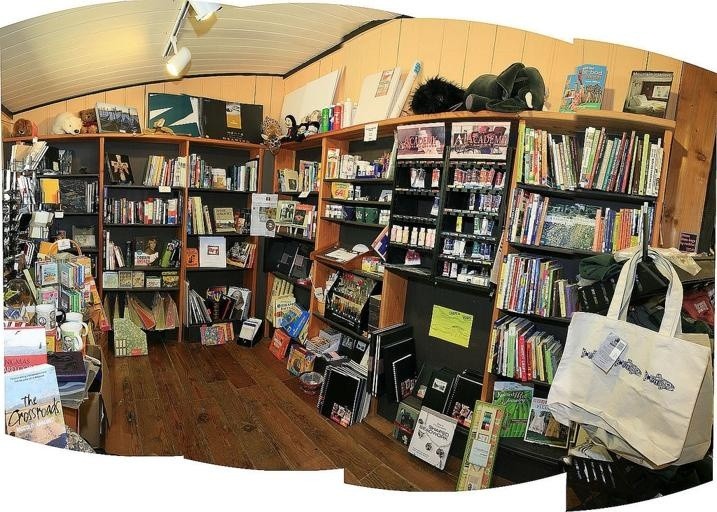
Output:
[93,101,141,135]
[355,68,403,121]
[556,74,582,114]
[620,70,673,120]
[570,64,606,111]
[2,348,92,451]
[383,79,403,119]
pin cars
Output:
[60,312,89,354]
[35,303,57,331]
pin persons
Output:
[270,115,298,151]
[306,120,320,138]
[292,122,308,139]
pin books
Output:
[186,281,251,323]
[105,153,187,186]
[355,68,403,121]
[266,277,375,427]
[366,124,511,471]
[383,79,403,119]
[507,187,656,253]
[187,197,251,234]
[2,348,92,451]
[493,381,571,449]
[186,236,256,268]
[93,101,141,135]
[515,123,664,197]
[570,64,606,111]
[620,70,673,120]
[103,271,180,288]
[250,194,278,237]
[488,315,562,384]
[103,187,183,224]
[267,149,396,277]
[103,228,181,271]
[496,254,613,318]
[556,74,582,114]
[187,154,259,192]
[3,142,98,313]
[200,318,262,346]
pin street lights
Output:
[546,308,713,512]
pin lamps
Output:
[161,0,222,77]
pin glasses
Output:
[161,0,222,77]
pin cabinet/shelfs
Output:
[2,133,268,342]
[269,110,678,484]
[321,97,358,133]
